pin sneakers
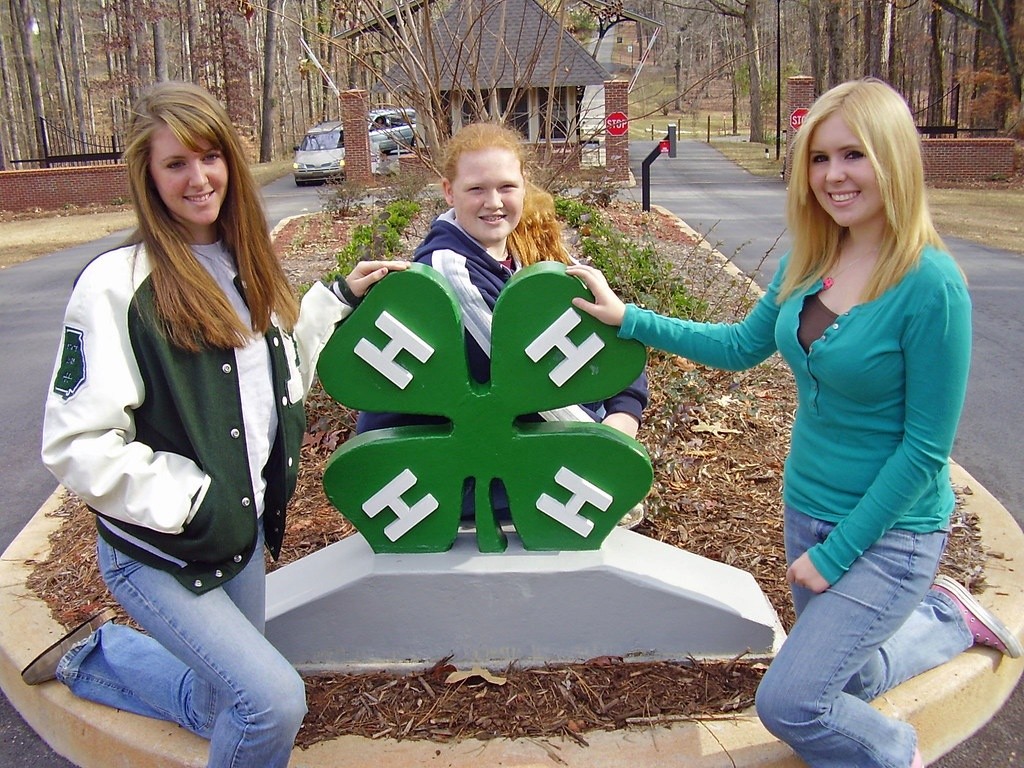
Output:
[910,747,924,768]
[930,575,1024,659]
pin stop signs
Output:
[605,112,629,137]
[790,108,810,133]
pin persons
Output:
[356,123,648,528]
[565,81,1021,768]
[22,83,412,768]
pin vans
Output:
[367,108,418,153]
[292,120,345,186]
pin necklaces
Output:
[822,247,878,291]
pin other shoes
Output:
[616,501,648,529]
[21,608,118,686]
